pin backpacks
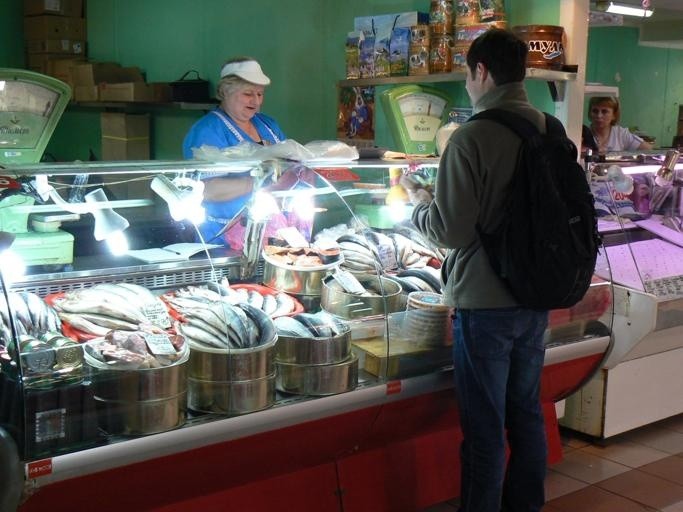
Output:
[465,108,605,312]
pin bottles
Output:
[650,150,680,213]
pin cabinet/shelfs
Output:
[2,149,617,507]
[557,150,683,448]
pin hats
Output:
[220,60,271,85]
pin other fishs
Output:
[335,223,445,292]
[62,282,173,331]
[0,274,350,368]
[0,285,61,336]
[173,275,342,353]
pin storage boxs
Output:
[25,1,166,102]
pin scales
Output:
[0,67,75,267]
[354,84,451,230]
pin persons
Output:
[407,28,605,512]
[182,56,286,258]
[582,97,652,156]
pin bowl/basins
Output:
[262,247,345,296]
[83,322,358,438]
[320,272,402,321]
